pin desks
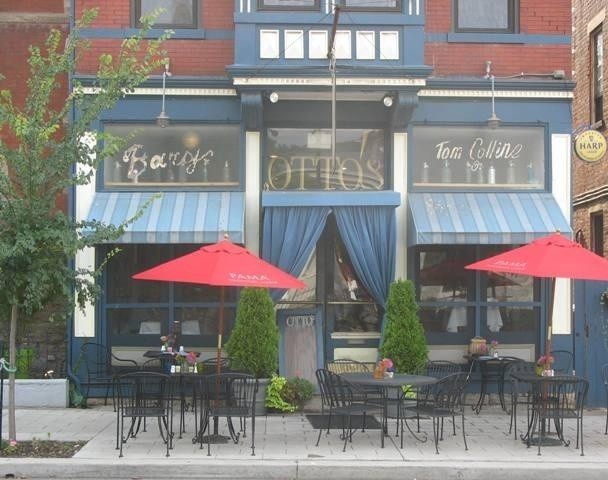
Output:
[463,355,524,415]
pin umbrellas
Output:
[463,227,607,440]
[130,234,310,435]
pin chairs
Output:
[81,342,138,412]
[507,350,590,456]
[602,363,608,435]
[315,358,473,454]
[116,350,259,457]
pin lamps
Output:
[382,93,394,108]
[485,61,501,130]
[265,92,278,103]
[156,57,171,128]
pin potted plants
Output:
[224,286,282,416]
[381,279,429,418]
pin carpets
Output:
[305,414,382,430]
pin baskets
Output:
[469,336,487,354]
